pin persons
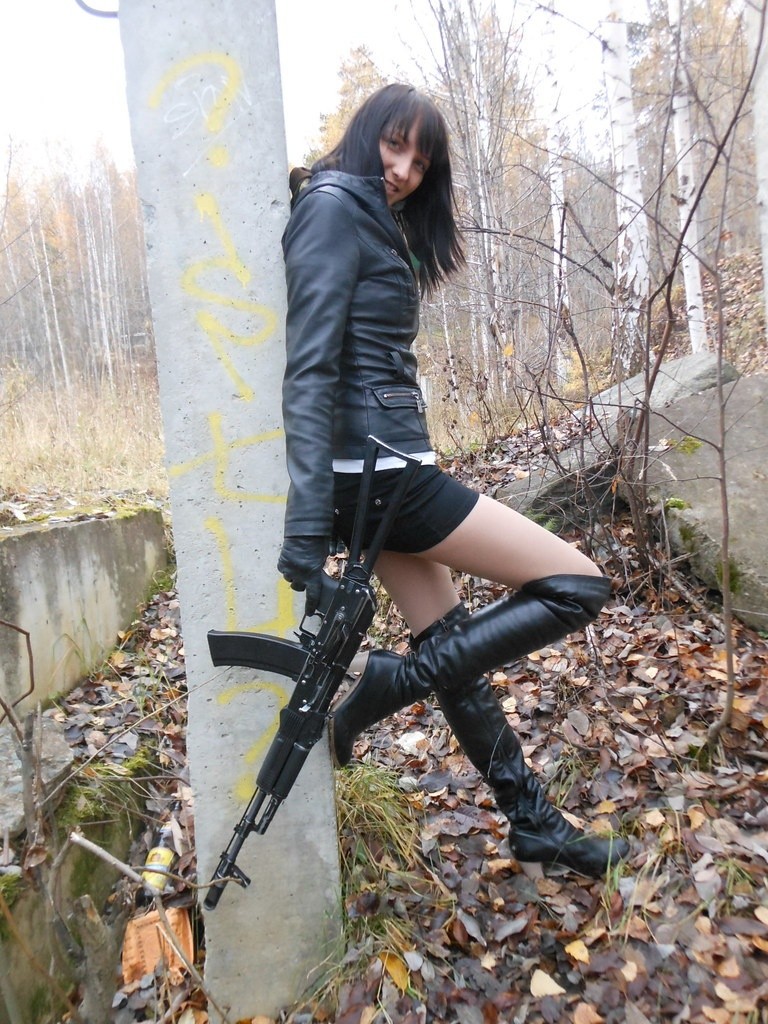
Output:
[276,86,628,870]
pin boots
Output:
[328,573,613,770]
[409,602,630,884]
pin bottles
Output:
[135,800,182,908]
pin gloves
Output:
[277,535,330,617]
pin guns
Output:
[203,431,421,913]
